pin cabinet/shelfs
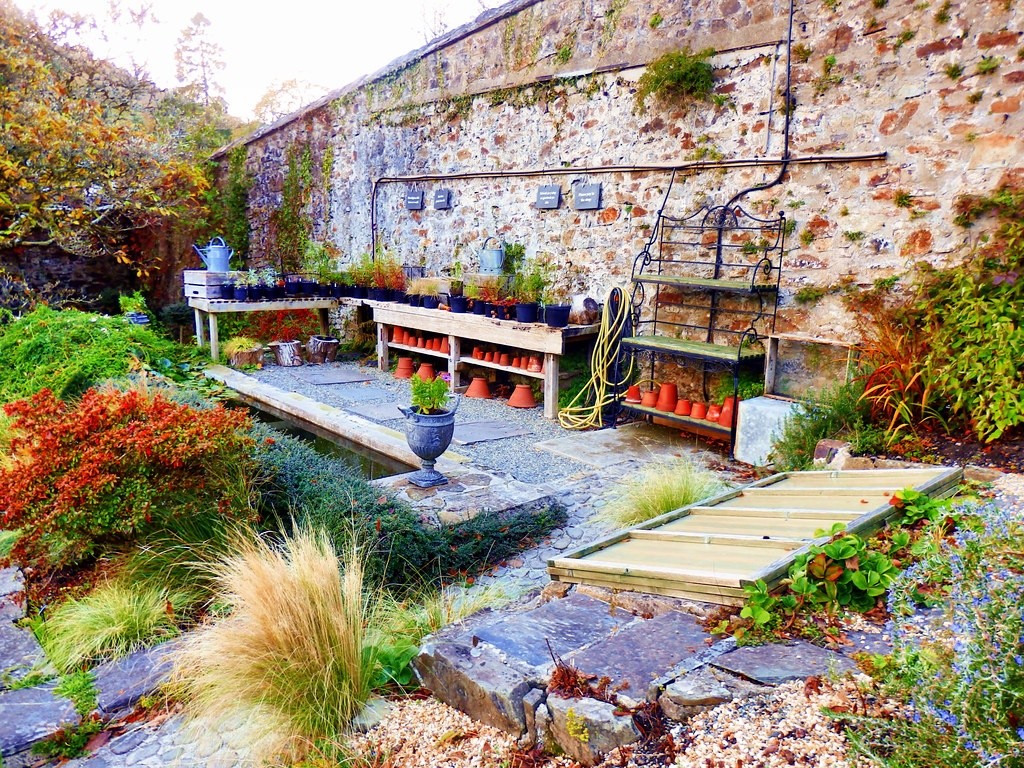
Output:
[365,300,600,418]
[600,204,789,463]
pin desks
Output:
[188,296,341,362]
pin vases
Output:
[624,383,743,429]
[392,324,544,409]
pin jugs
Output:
[467,236,505,276]
[192,236,234,273]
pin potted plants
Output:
[118,288,159,330]
[158,303,194,345]
[398,372,462,488]
[222,243,573,327]
